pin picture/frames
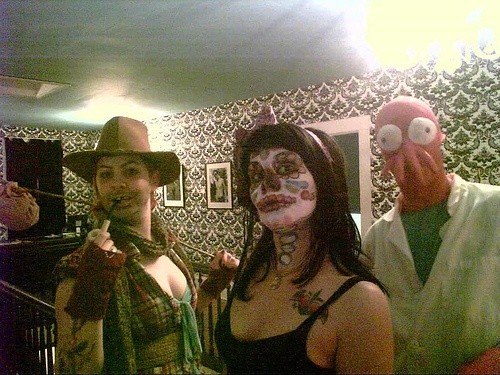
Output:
[205,161,233,210]
[162,164,184,208]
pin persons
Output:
[54,117,241,375]
[357,94,500,375]
[216,113,395,375]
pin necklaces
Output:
[272,260,308,290]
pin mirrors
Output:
[298,114,372,248]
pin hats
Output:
[61,117,181,186]
